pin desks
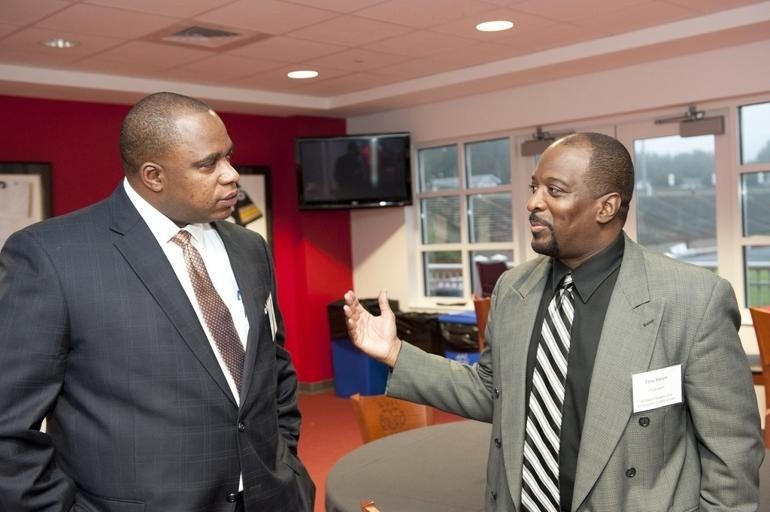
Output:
[323,390,580,509]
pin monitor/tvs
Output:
[295,131,413,211]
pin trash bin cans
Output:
[327,298,481,400]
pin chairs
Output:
[345,391,437,448]
[748,305,770,453]
[474,290,498,363]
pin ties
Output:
[520,273,575,511]
[171,231,245,399]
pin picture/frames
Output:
[226,160,278,257]
[0,157,57,225]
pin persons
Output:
[0,92,316,512]
[343,133,766,512]
